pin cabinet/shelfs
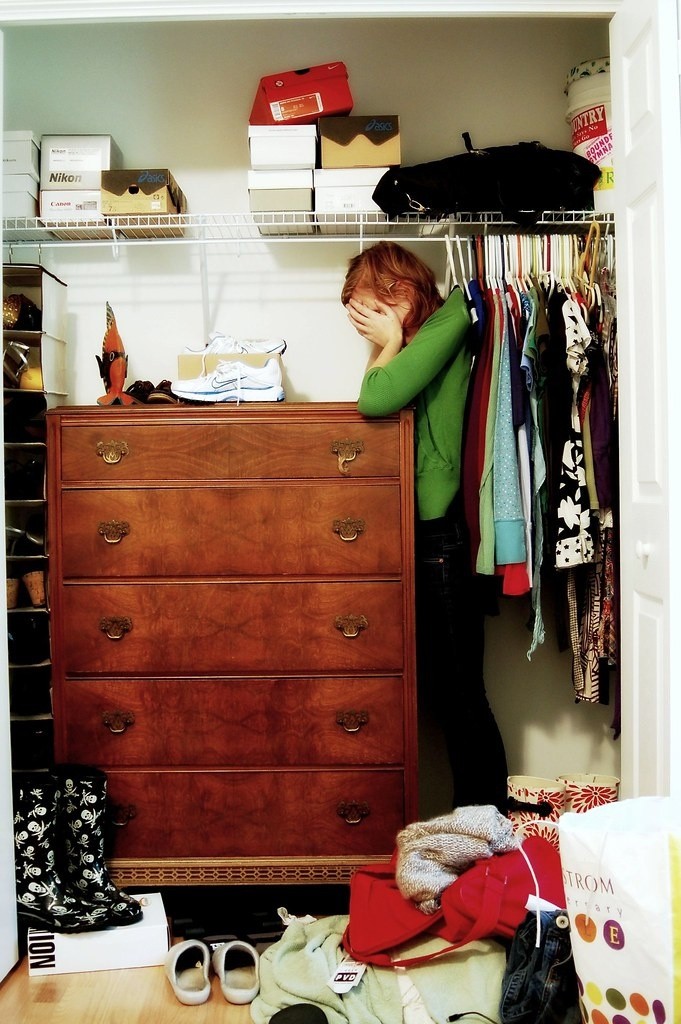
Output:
[43,399,421,888]
[3,259,66,778]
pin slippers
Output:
[212,941,259,1005]
[164,940,211,1005]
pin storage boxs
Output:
[246,170,314,235]
[29,891,171,977]
[3,128,188,236]
[246,125,318,170]
[316,114,403,169]
[177,351,285,389]
[314,168,392,231]
[247,60,353,125]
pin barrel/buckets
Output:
[563,57,615,214]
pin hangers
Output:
[445,218,615,325]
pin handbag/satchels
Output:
[371,132,602,225]
[558,796,681,1024]
[342,835,567,967]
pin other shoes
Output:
[3,293,57,770]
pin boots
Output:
[49,763,143,926]
[12,771,111,934]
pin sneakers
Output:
[184,334,286,357]
[170,357,284,407]
[124,380,155,404]
[148,379,180,403]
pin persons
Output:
[341,241,509,817]
